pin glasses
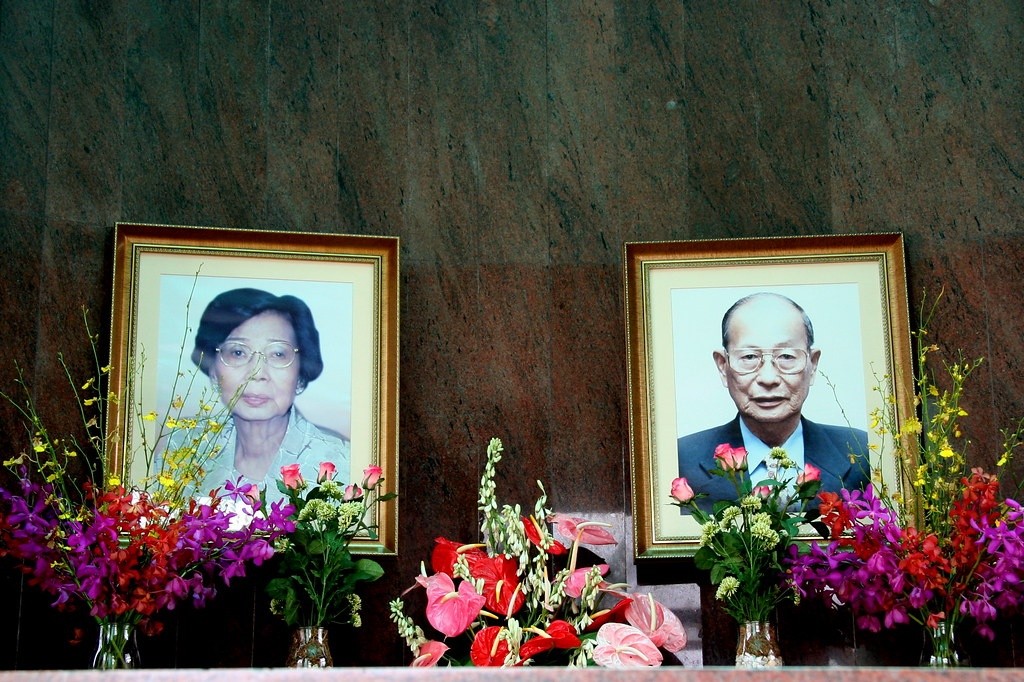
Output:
[215,341,300,368]
[722,347,810,375]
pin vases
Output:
[286,626,333,668]
[734,620,782,667]
[92,622,140,669]
[918,620,960,667]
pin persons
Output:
[154,287,351,526]
[677,290,870,527]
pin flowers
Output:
[266,460,397,627]
[668,443,820,618]
[774,343,1024,640]
[387,434,686,669]
[0,260,291,615]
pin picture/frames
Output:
[98,220,400,557]
[621,231,925,564]
